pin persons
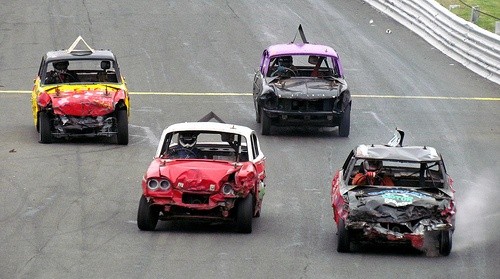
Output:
[45,60,80,84]
[352,159,395,186]
[169,132,205,159]
[272,56,300,77]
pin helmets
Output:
[53,60,69,73]
[278,56,293,68]
[364,158,383,171]
[178,131,197,148]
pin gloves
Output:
[53,72,59,80]
[169,148,178,156]
[367,171,376,178]
[278,66,284,72]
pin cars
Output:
[331,127,456,255]
[137,111,266,233]
[253,24,351,136]
[32,35,130,145]
